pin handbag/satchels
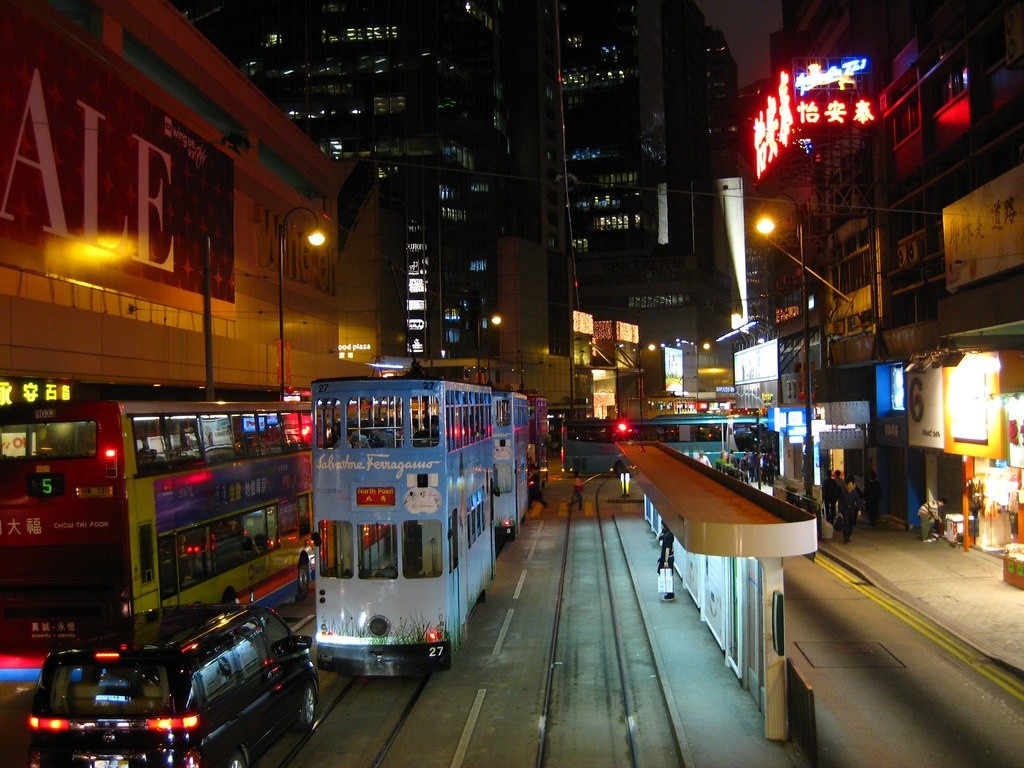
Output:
[657,565,674,593]
[834,516,844,531]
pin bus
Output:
[491,389,533,540]
[528,394,550,505]
[0,400,396,685]
[310,376,496,676]
[560,412,781,479]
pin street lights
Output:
[476,313,503,384]
[697,341,710,414]
[758,193,818,494]
[278,205,324,400]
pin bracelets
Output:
[664,560,668,562]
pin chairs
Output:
[160,532,271,594]
[139,448,195,465]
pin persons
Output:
[527,464,548,512]
[821,470,883,544]
[326,415,439,449]
[656,520,674,602]
[696,451,712,468]
[727,444,774,486]
[917,497,946,542]
[567,470,585,511]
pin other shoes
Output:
[923,537,936,543]
[662,595,675,602]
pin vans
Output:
[24,603,318,768]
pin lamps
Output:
[904,350,964,373]
